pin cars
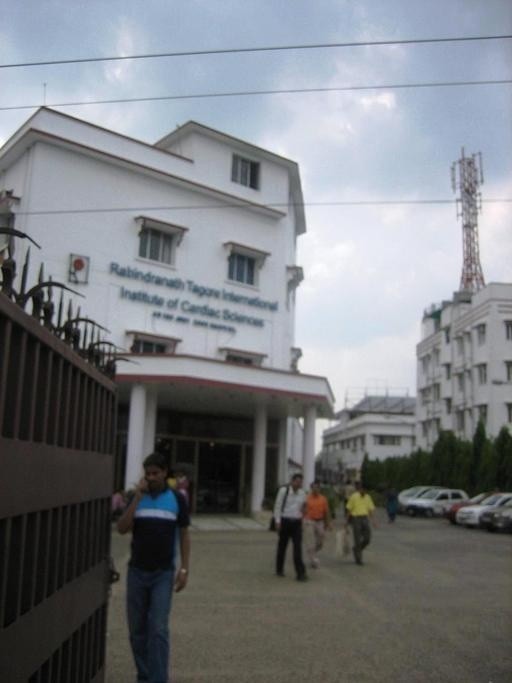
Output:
[398,485,511,531]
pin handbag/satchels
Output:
[269,518,276,532]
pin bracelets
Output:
[179,568,189,575]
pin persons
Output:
[118,454,190,683]
[273,473,399,582]
[176,470,189,502]
[166,471,178,490]
